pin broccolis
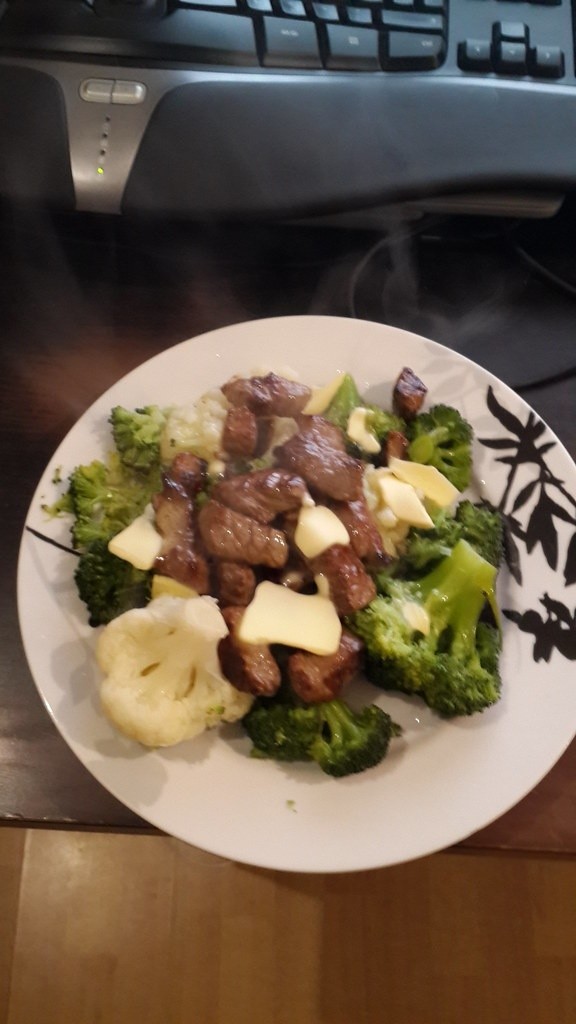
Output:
[64,375,506,773]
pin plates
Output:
[17,315,576,873]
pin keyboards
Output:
[0,0,576,224]
